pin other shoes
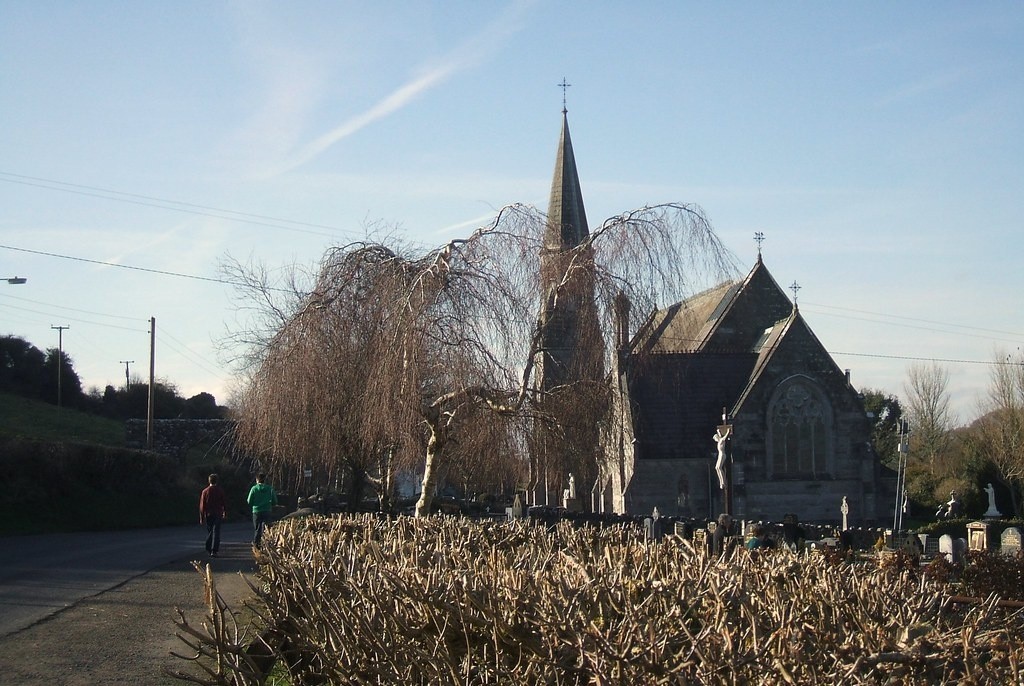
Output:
[250,540,261,547]
[208,551,219,558]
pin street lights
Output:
[894,416,904,529]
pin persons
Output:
[984,484,999,514]
[568,473,576,498]
[199,474,225,558]
[712,428,730,490]
[712,513,733,557]
[247,474,278,549]
[752,527,777,555]
